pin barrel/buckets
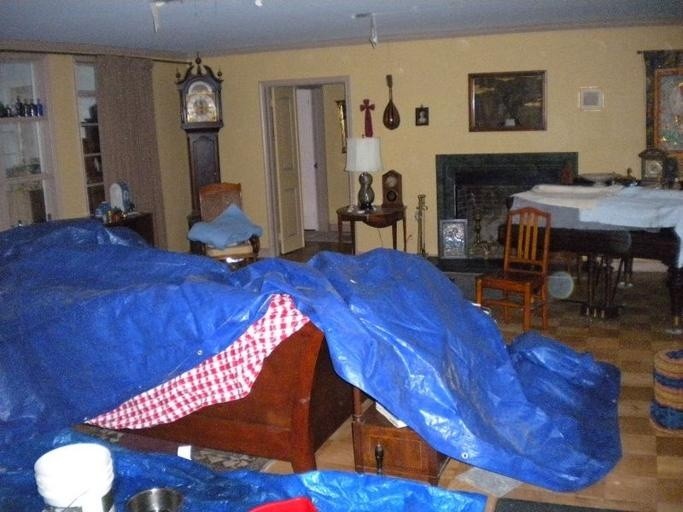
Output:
[35,443,115,512]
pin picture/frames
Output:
[438,218,470,260]
[578,85,606,112]
[467,69,547,133]
[416,107,429,126]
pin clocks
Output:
[175,53,225,131]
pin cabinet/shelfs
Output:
[643,49,682,179]
[0,51,60,231]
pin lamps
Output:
[345,137,382,209]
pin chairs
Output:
[475,206,553,336]
[189,181,260,265]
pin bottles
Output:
[1,95,43,118]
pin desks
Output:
[102,210,155,248]
[336,203,408,255]
[506,184,682,331]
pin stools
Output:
[650,347,683,439]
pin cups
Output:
[36,444,184,512]
